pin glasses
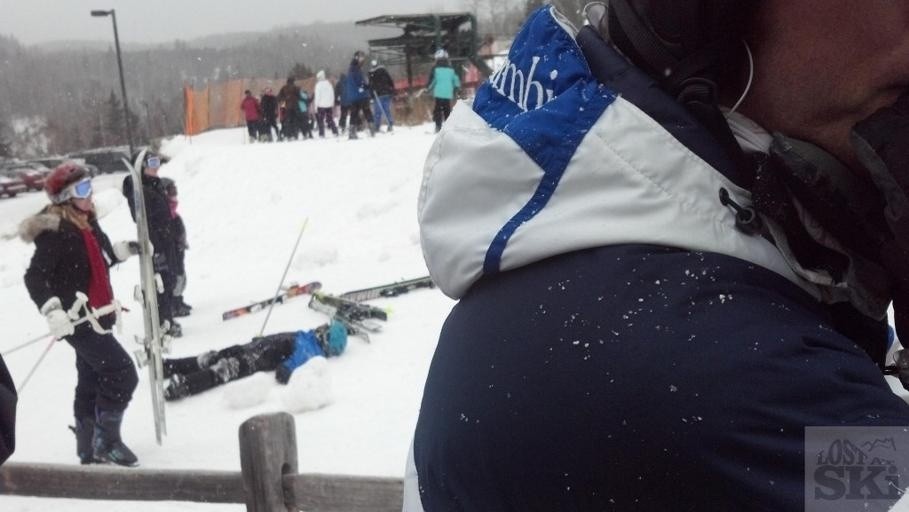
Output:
[57,176,93,203]
[148,157,160,168]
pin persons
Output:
[0,2,909,512]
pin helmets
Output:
[130,150,161,172]
[322,320,348,357]
[45,164,89,204]
[435,50,448,59]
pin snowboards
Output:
[341,276,432,303]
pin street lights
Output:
[89,5,137,173]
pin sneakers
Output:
[144,341,172,379]
[165,374,190,401]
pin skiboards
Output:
[223,280,321,320]
[309,289,388,341]
[120,148,170,444]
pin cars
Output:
[0,143,169,202]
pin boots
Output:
[348,122,376,139]
[91,407,139,467]
[159,296,191,336]
[74,416,100,464]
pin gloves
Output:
[771,107,909,391]
[276,364,291,384]
[110,239,140,263]
[40,298,74,340]
